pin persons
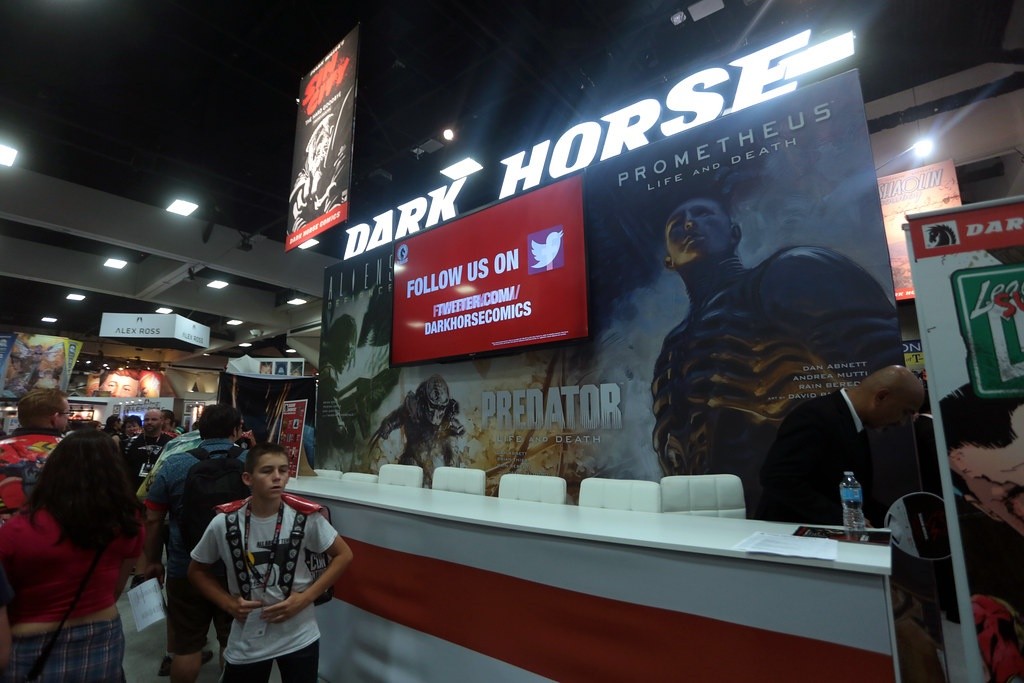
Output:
[89,405,205,505]
[757,364,925,522]
[98,371,139,398]
[124,407,174,592]
[651,194,904,480]
[187,441,353,683]
[0,389,75,532]
[143,402,251,683]
[0,429,142,683]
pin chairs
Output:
[432,467,485,495]
[313,469,342,479]
[498,473,566,504]
[578,477,661,513]
[378,463,423,487]
[660,474,746,520]
[341,472,378,483]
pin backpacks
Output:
[177,445,252,573]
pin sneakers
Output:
[158,650,213,675]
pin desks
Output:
[284,474,899,682]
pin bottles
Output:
[840,471,865,536]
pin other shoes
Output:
[130,574,164,590]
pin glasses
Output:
[49,410,74,417]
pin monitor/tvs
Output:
[389,166,593,367]
